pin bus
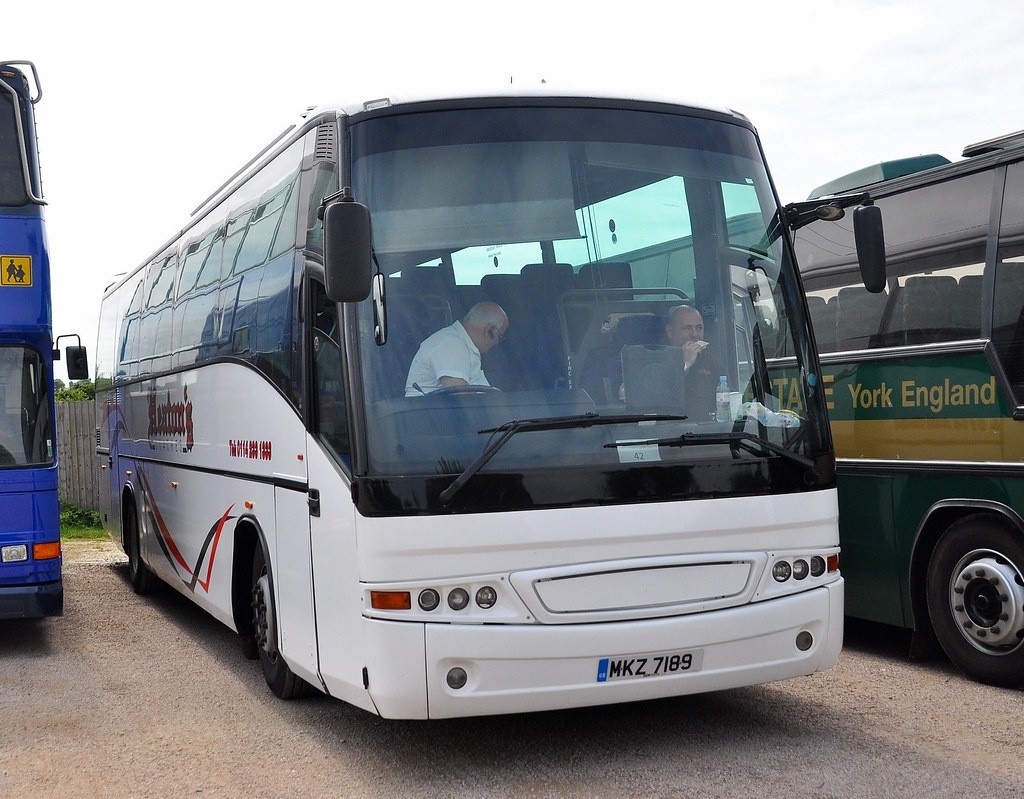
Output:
[0,59,89,628]
[93,88,887,721]
[580,129,1024,686]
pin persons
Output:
[611,304,732,422]
[404,300,509,398]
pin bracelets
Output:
[683,362,689,374]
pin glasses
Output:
[495,326,507,343]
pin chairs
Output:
[380,252,638,394]
[750,262,1023,383]
